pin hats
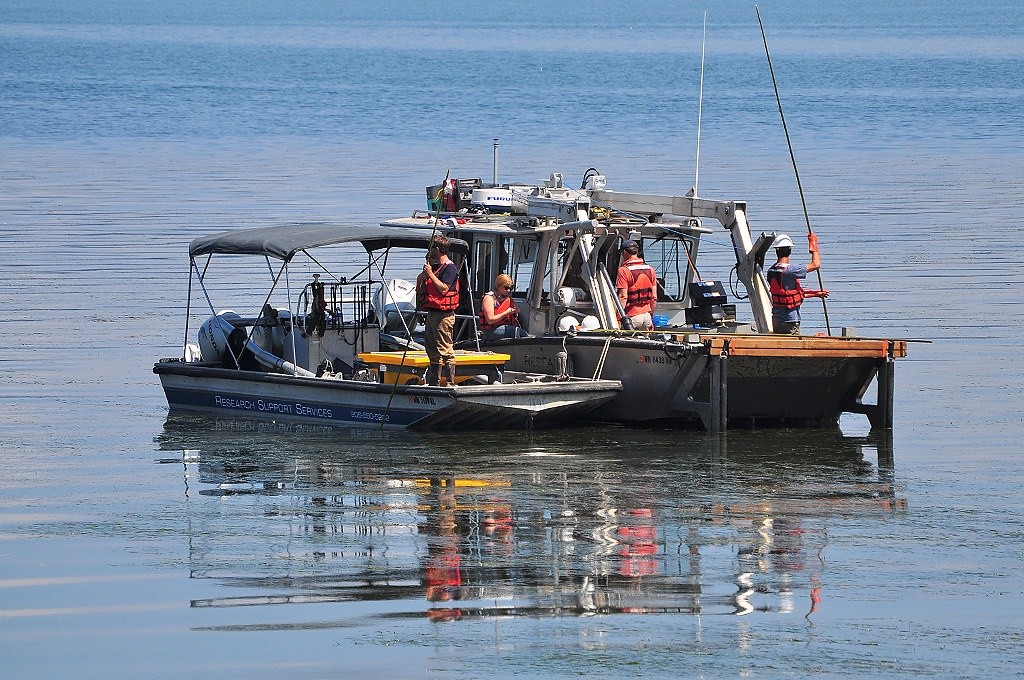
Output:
[617,240,639,252]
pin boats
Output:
[152,222,625,433]
[379,6,909,434]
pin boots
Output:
[429,363,442,386]
[445,363,455,387]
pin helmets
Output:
[772,234,795,247]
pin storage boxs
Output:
[359,351,510,384]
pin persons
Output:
[485,236,509,293]
[479,274,531,339]
[767,234,830,335]
[415,235,459,390]
[615,240,657,333]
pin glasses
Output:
[503,285,512,292]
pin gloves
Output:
[649,323,654,331]
[808,232,819,254]
[615,308,621,330]
[809,290,829,299]
